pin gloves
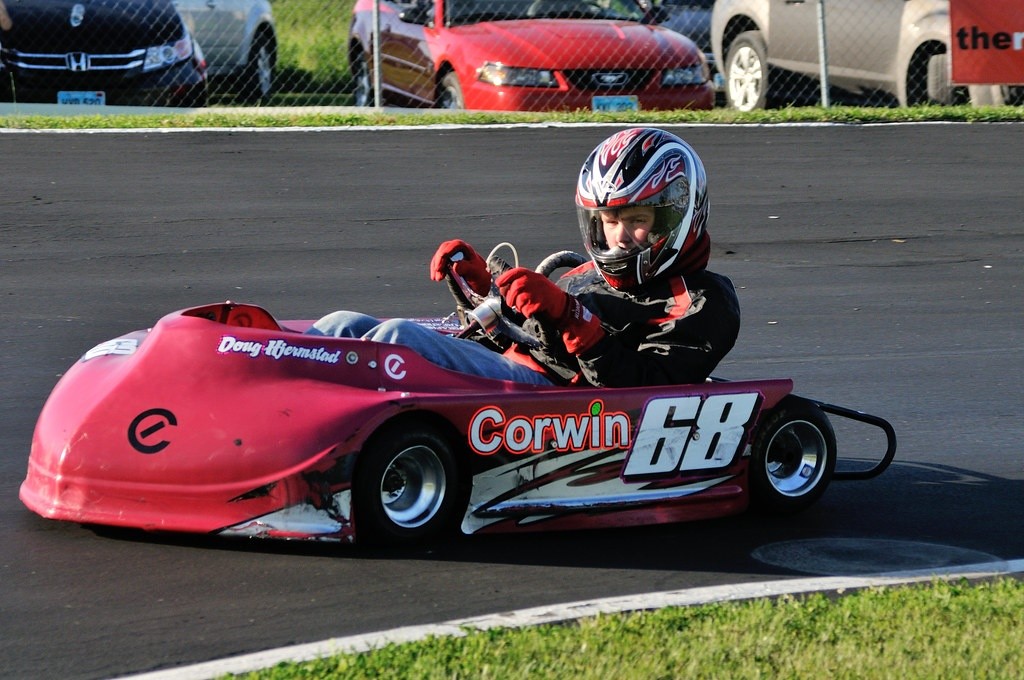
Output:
[495,267,605,357]
[429,238,492,297]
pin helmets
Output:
[576,127,710,292]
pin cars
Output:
[0,0,210,109]
[639,0,864,109]
[711,0,1024,110]
[170,0,278,107]
[348,0,717,113]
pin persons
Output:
[306,126,740,390]
